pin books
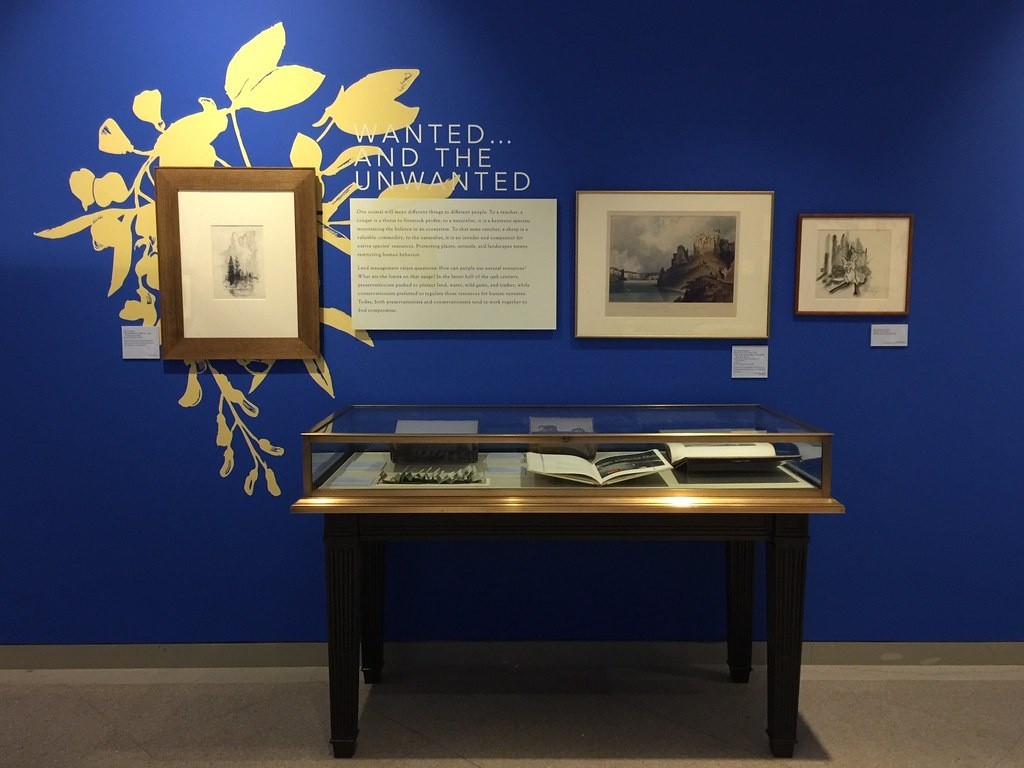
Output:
[527,446,674,485]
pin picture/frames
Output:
[793,213,914,315]
[155,166,320,360]
[575,189,775,338]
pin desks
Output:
[291,405,845,757]
